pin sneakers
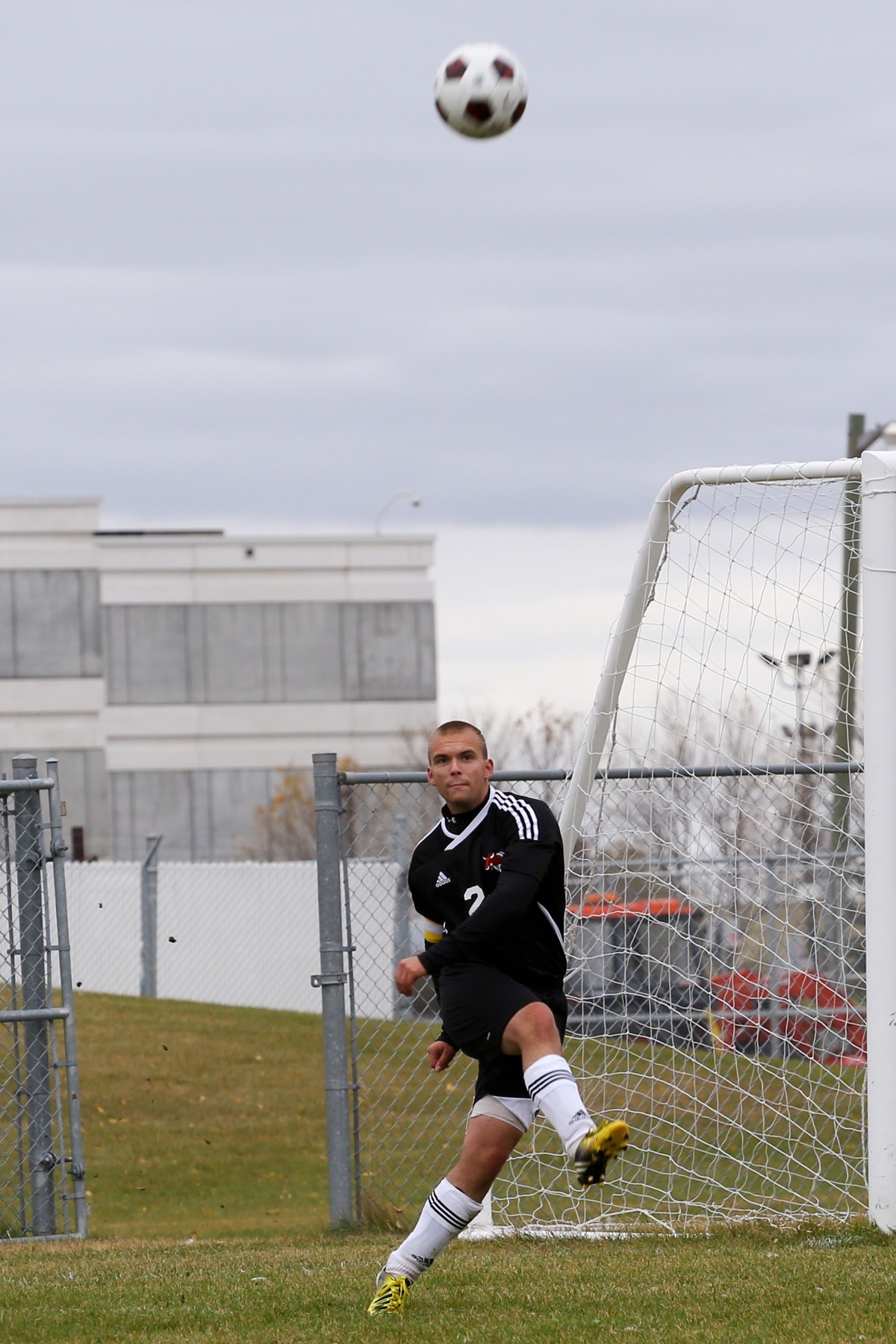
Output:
[366,1264,414,1326]
[573,1119,631,1189]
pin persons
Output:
[367,720,632,1317]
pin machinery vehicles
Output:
[543,888,865,1076]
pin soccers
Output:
[434,35,529,139]
